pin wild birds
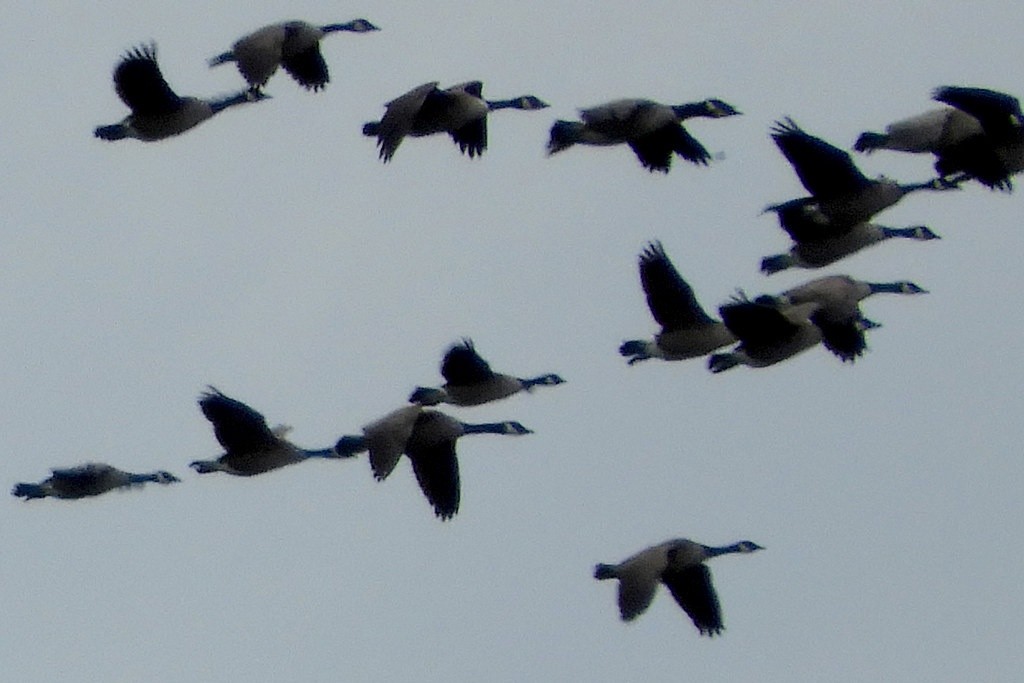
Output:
[616,236,930,373]
[207,18,382,95]
[93,38,272,144]
[362,77,554,165]
[10,462,180,503]
[594,536,768,639]
[190,385,358,477]
[409,339,569,406]
[337,402,535,521]
[544,96,748,175]
[760,82,1023,275]
